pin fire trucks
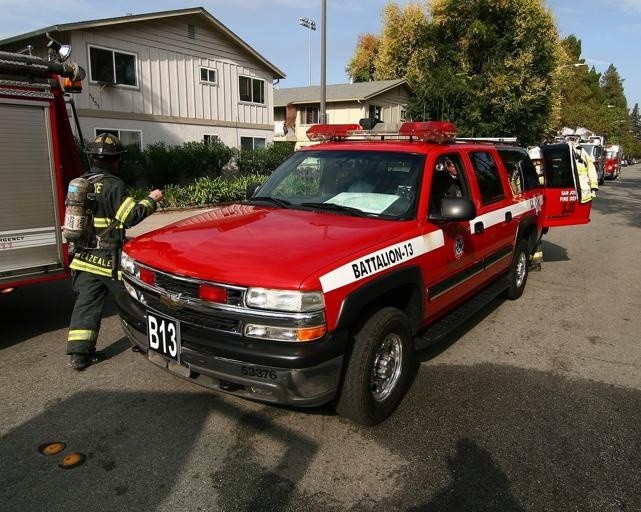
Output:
[0,41,92,293]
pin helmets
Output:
[82,132,128,156]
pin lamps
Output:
[47,39,72,61]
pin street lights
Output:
[298,16,316,87]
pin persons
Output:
[66,134,163,371]
[435,159,457,180]
[510,141,599,272]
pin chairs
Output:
[349,168,376,192]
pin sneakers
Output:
[70,346,107,370]
